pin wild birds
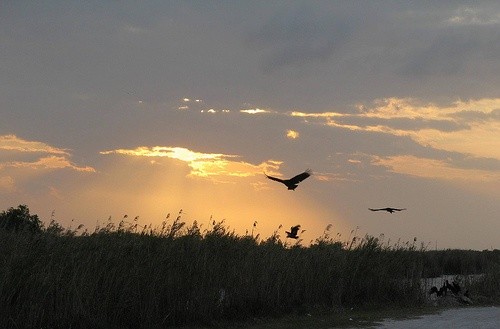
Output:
[285,225,301,239]
[264,167,314,191]
[368,207,408,214]
